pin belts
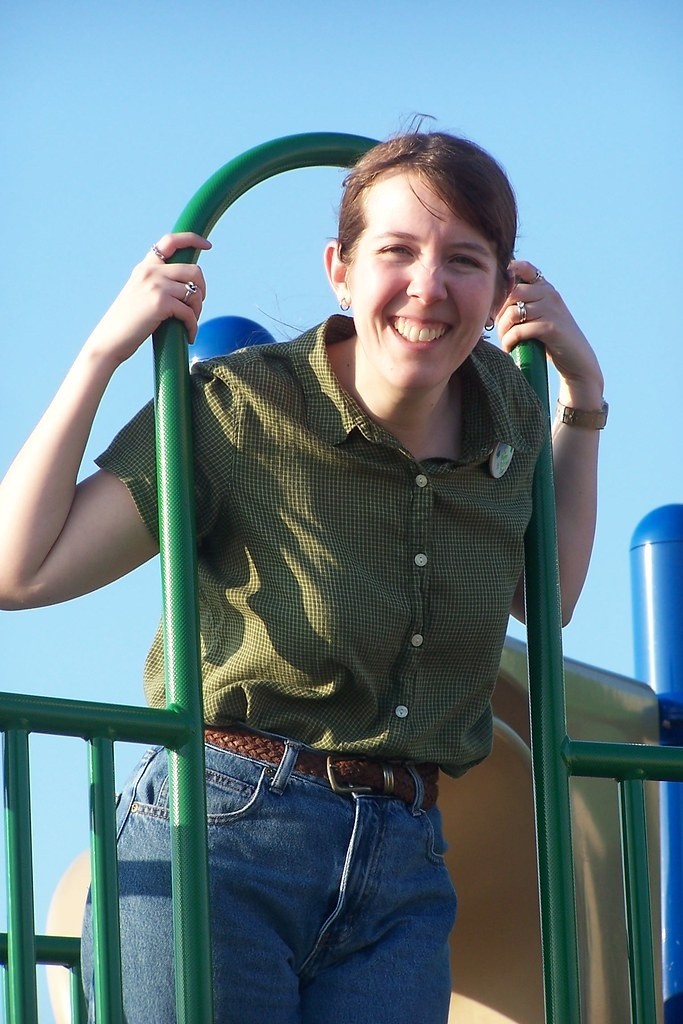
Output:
[203,726,441,810]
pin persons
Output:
[0,112,604,1024]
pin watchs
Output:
[556,398,609,428]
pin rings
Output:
[529,269,544,283]
[516,300,527,324]
[181,282,197,302]
[152,244,166,260]
